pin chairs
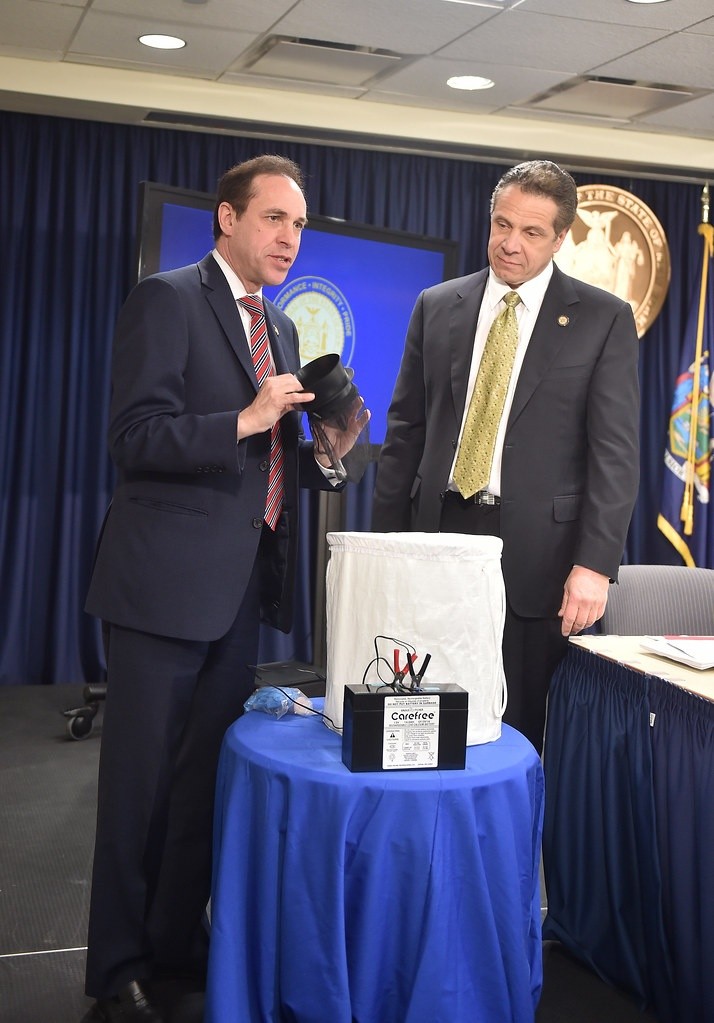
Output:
[600,565,714,636]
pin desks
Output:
[205,698,545,1023]
[542,635,713,1023]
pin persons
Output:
[371,157,642,758]
[84,157,369,1023]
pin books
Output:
[643,635,714,671]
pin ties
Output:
[453,292,521,500]
[237,296,284,531]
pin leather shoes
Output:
[95,980,167,1023]
[146,960,206,983]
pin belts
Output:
[445,489,500,506]
